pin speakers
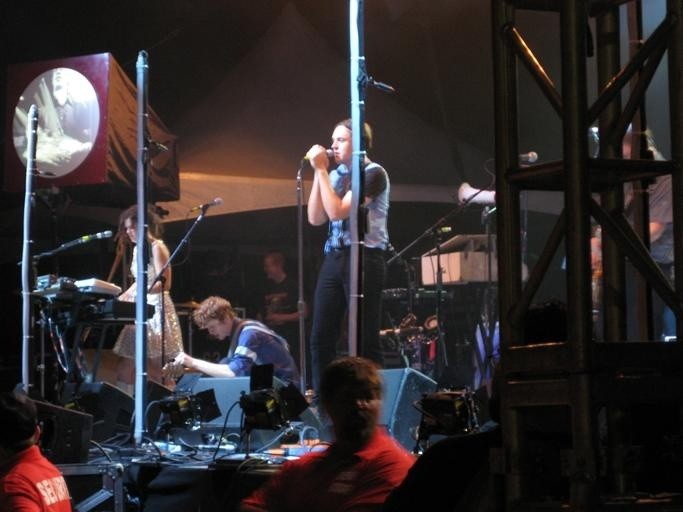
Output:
[61,381,136,450]
[33,399,94,463]
[143,379,173,421]
[375,369,437,449]
[179,375,318,445]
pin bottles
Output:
[138,441,182,453]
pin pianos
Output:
[421,234,529,287]
[37,303,156,326]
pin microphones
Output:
[518,151,539,164]
[308,149,334,160]
[89,230,112,240]
[194,197,222,210]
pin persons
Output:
[238,355,422,510]
[381,359,572,512]
[0,391,73,512]
[306,119,391,396]
[185,227,262,361]
[115,204,185,399]
[589,122,678,343]
[161,296,301,397]
[255,251,309,374]
